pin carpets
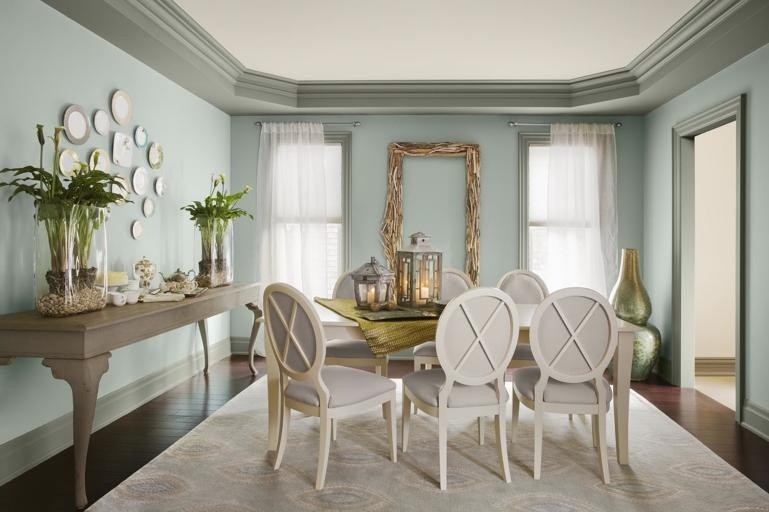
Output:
[83,373,767,512]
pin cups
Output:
[160,282,197,294]
[107,280,139,306]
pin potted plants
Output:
[0,123,136,316]
[177,170,254,288]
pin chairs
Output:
[262,280,399,490]
[411,268,475,420]
[401,287,520,491]
[497,269,541,380]
[511,285,619,486]
[321,270,391,441]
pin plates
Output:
[58,88,167,241]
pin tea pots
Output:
[132,255,159,290]
[158,268,197,281]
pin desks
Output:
[307,296,643,465]
[1,282,264,512]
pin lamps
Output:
[349,256,393,311]
[393,228,443,308]
